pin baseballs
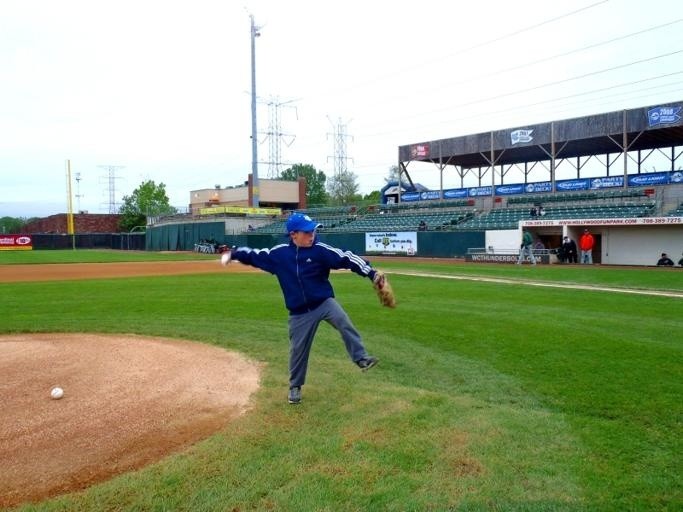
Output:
[221,253,230,264]
[51,387,63,399]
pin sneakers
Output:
[356,357,379,371]
[288,387,301,404]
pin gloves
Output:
[221,250,231,266]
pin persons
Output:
[529,208,545,217]
[533,240,545,256]
[516,230,536,264]
[578,228,594,264]
[560,236,577,263]
[418,221,428,231]
[656,252,673,265]
[220,212,395,404]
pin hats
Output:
[286,212,324,232]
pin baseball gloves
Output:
[371,270,396,308]
